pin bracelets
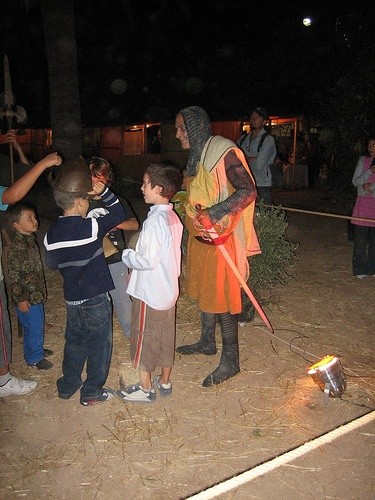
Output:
[16,145,22,152]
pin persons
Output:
[349,136,375,279]
[43,170,128,405]
[169,105,262,387]
[0,128,63,337]
[114,163,184,403]
[86,156,140,340]
[151,136,163,154]
[8,199,55,369]
[0,151,62,400]
[235,107,278,221]
[271,116,375,241]
[0,130,40,203]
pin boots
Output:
[175,310,219,355]
[200,310,241,387]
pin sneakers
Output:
[0,374,38,399]
[80,388,116,405]
[153,374,172,395]
[116,383,156,403]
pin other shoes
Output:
[357,274,368,279]
[30,359,53,370]
[42,349,53,357]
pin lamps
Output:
[307,356,347,398]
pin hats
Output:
[54,154,92,193]
[248,107,270,119]
